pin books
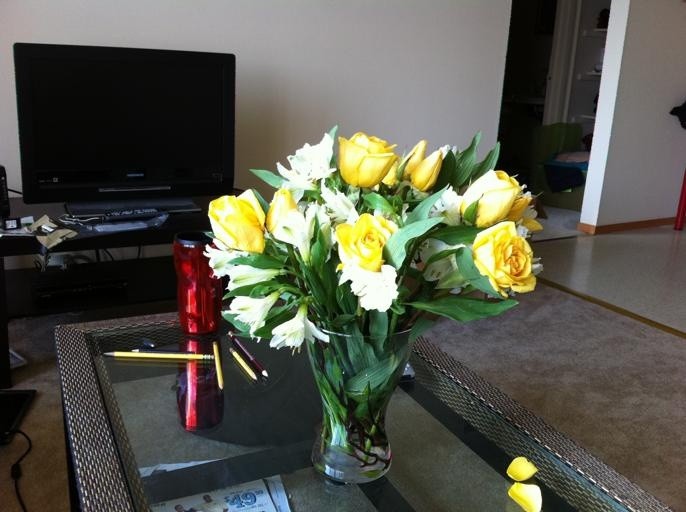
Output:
[137,458,292,512]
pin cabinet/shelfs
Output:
[1,217,219,317]
[540,0,612,191]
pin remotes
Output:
[104,208,157,221]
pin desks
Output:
[51,312,681,512]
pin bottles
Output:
[176,339,225,433]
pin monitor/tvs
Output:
[13,42,236,218]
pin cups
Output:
[168,232,223,338]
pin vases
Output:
[298,313,412,485]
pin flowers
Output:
[188,127,544,462]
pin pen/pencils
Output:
[102,331,269,392]
